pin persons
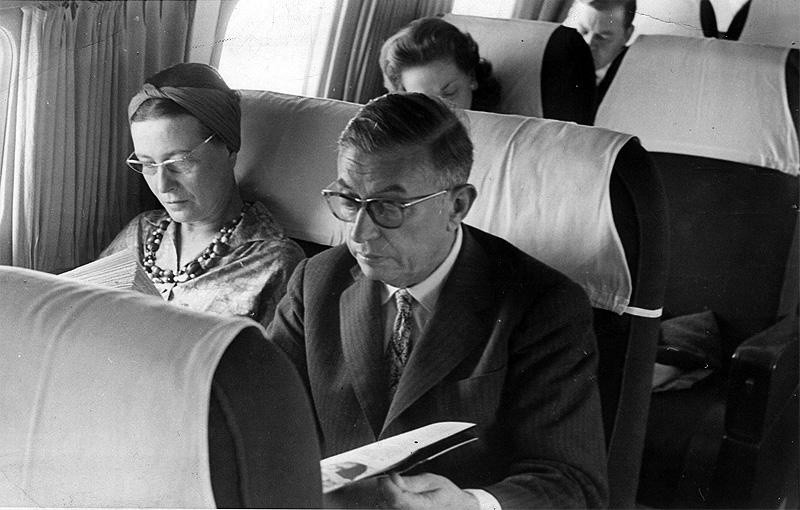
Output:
[268,93,611,510]
[98,63,307,330]
[580,0,636,86]
[378,16,501,112]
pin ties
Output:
[380,288,414,403]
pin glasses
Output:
[320,179,447,228]
[126,134,216,175]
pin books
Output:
[319,421,479,494]
[56,245,163,297]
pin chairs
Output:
[2,1,800,509]
